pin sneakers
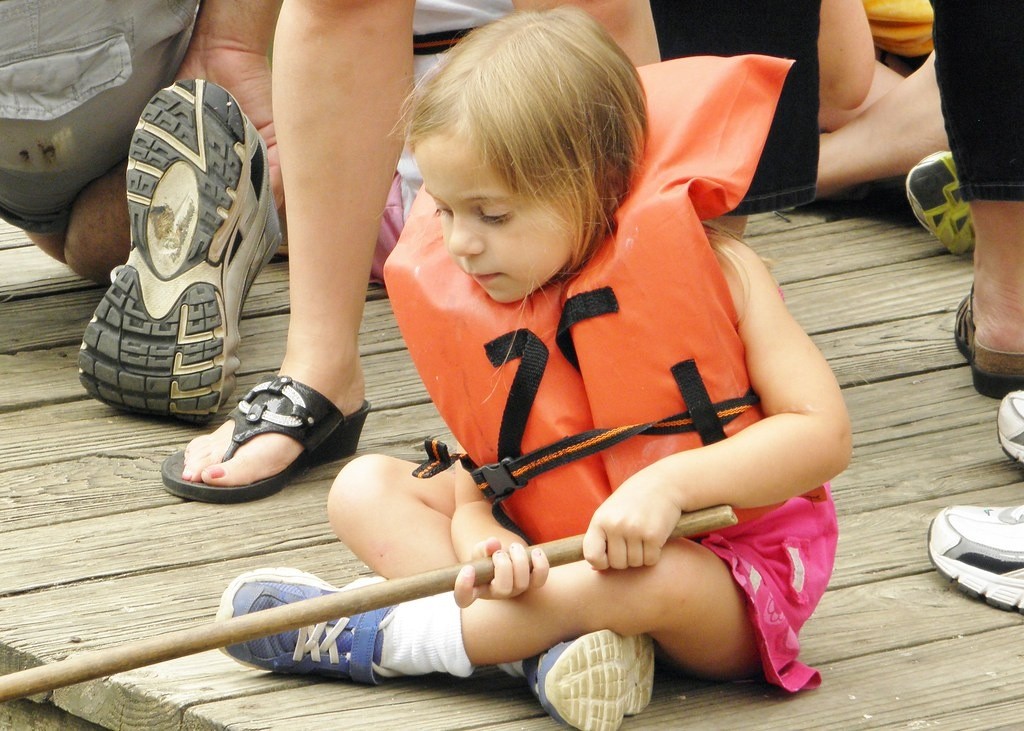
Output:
[524,627,656,730]
[928,506,1023,615]
[997,389,1024,464]
[79,78,281,429]
[219,569,400,687]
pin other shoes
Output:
[905,150,975,257]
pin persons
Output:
[0,1,1024,614]
[212,6,855,731]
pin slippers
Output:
[954,281,1024,399]
[159,373,371,505]
